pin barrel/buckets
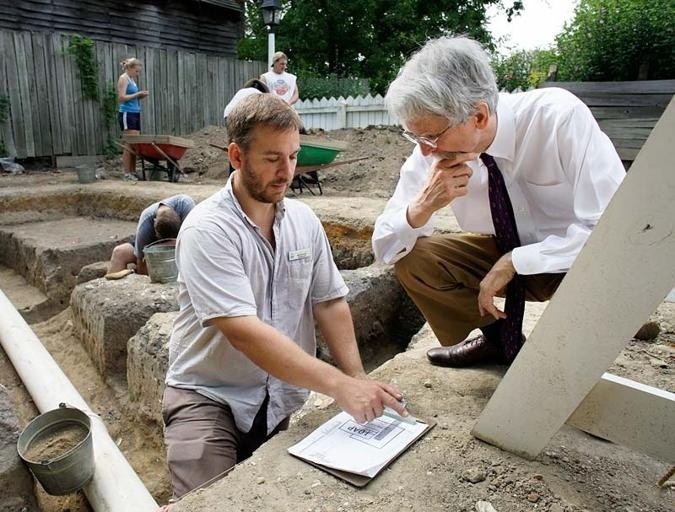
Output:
[15,402,97,496]
[76,165,96,185]
[141,240,180,284]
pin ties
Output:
[479,151,526,362]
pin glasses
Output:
[402,123,453,148]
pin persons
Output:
[223,79,269,175]
[163,94,408,500]
[116,56,147,180]
[371,36,629,364]
[107,194,195,276]
[258,53,299,108]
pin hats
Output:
[271,51,286,67]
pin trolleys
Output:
[212,137,345,197]
[120,129,194,183]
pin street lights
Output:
[258,0,283,72]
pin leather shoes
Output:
[426,334,526,368]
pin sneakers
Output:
[124,174,139,181]
